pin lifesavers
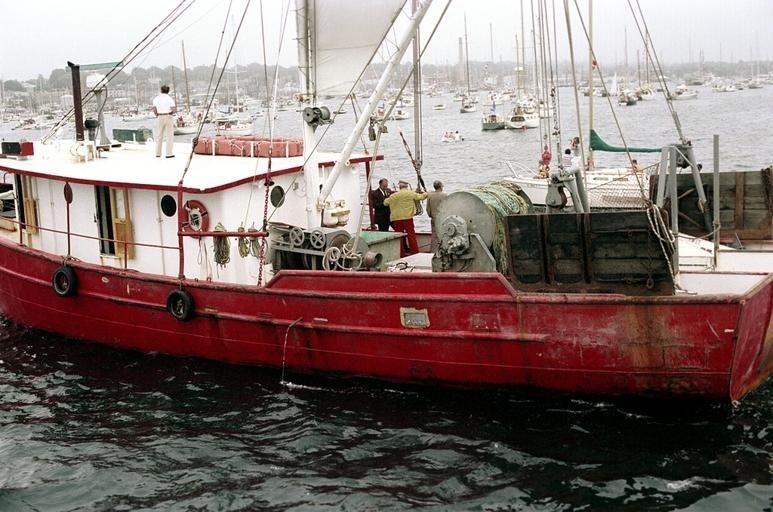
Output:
[182,200,209,238]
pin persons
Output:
[454,131,460,142]
[443,131,449,139]
[371,177,410,253]
[426,179,446,253]
[569,137,581,157]
[150,85,176,159]
[559,147,572,166]
[381,182,427,259]
[449,131,454,141]
[630,159,640,173]
